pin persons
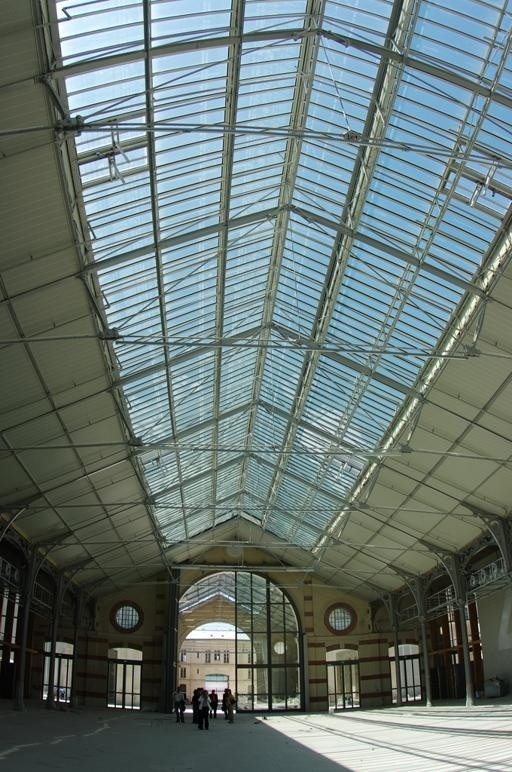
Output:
[172,687,235,730]
